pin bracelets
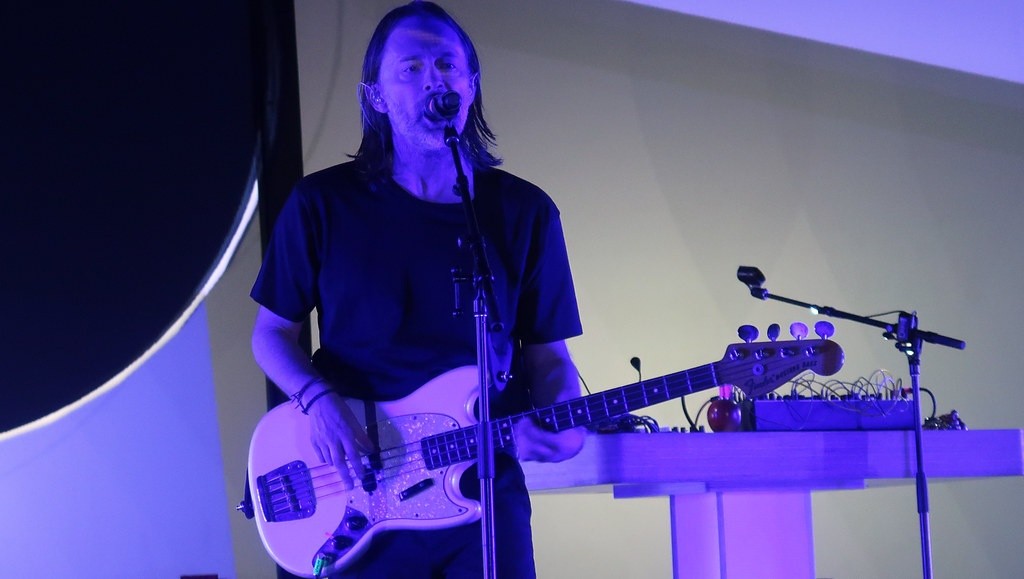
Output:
[290,373,337,415]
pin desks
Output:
[519,429,1023,579]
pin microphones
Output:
[423,91,462,123]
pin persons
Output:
[249,0,586,579]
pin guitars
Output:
[247,321,845,579]
